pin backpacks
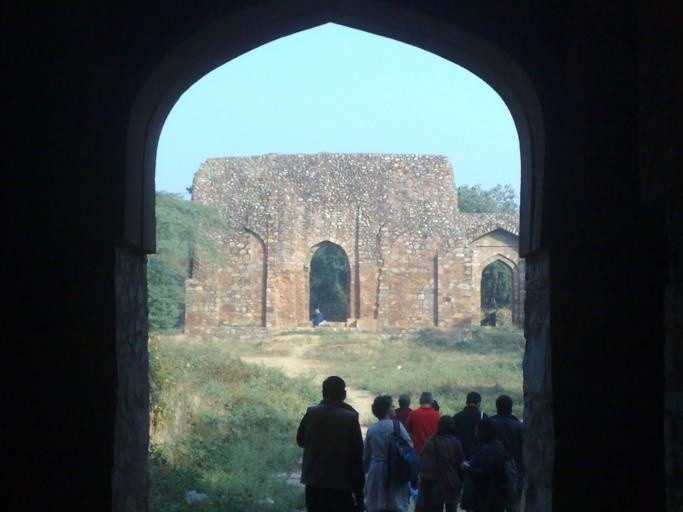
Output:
[387,423,419,488]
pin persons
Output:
[405,391,442,499]
[409,415,465,511]
[394,395,414,429]
[431,400,441,416]
[470,394,524,512]
[295,375,367,512]
[311,308,325,327]
[359,394,415,512]
[459,452,521,511]
[451,391,489,510]
